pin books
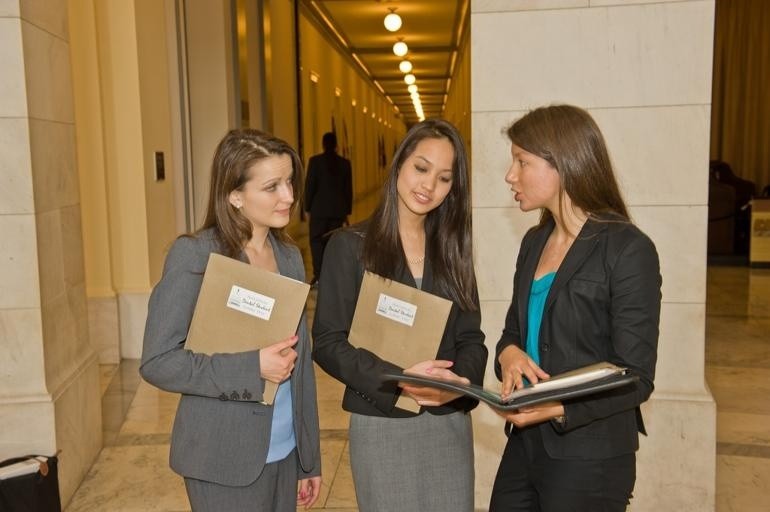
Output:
[386,360,639,411]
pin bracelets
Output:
[555,416,567,425]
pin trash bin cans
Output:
[0,455,62,512]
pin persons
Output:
[138,126,324,512]
[302,131,353,288]
[488,102,664,512]
[311,116,488,512]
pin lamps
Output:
[382,7,426,123]
